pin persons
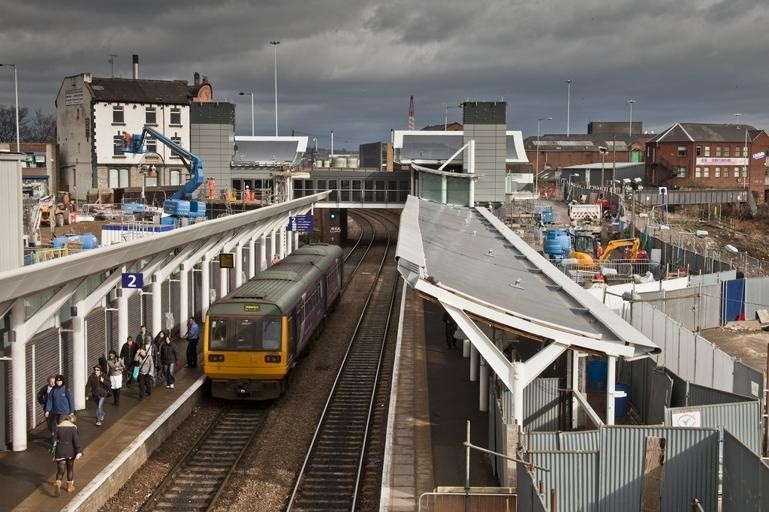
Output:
[62,191,71,204]
[35,315,201,498]
[442,308,458,350]
[487,199,495,212]
[206,174,218,199]
[68,199,79,224]
[220,188,236,218]
[237,184,256,201]
[596,239,604,258]
[122,129,133,148]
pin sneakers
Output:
[165,383,175,389]
[95,420,102,426]
[100,412,105,421]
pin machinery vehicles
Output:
[122,125,209,226]
[39,191,76,229]
[538,193,665,280]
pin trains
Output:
[200,239,344,404]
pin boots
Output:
[54,479,62,496]
[115,390,119,406]
[65,480,75,493]
[112,391,117,406]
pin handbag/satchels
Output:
[103,384,113,398]
[132,366,140,383]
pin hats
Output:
[54,375,64,383]
[93,364,102,369]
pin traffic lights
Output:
[330,213,336,220]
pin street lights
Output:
[535,117,553,196]
[598,146,609,192]
[565,78,574,138]
[269,40,282,136]
[312,137,318,152]
[0,64,20,153]
[733,112,742,123]
[623,176,644,239]
[627,96,638,138]
[568,172,579,190]
[237,92,255,136]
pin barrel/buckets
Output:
[587,358,607,395]
[608,383,631,418]
